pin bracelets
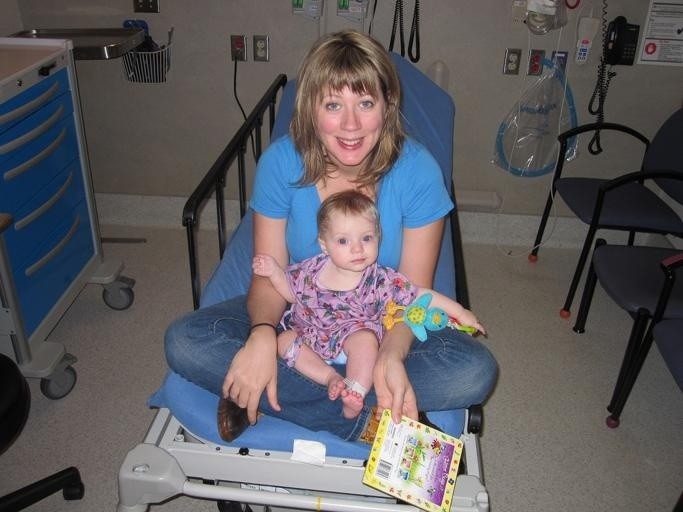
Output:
[249,322,278,334]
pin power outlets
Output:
[551,51,567,72]
[502,49,521,75]
[528,50,545,76]
[231,35,246,61]
[253,35,269,62]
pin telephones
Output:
[604,16,640,65]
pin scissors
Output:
[123,20,148,37]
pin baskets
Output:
[122,43,172,85]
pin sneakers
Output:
[217,398,250,442]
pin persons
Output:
[164,29,499,477]
[251,188,488,420]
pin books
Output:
[362,408,464,512]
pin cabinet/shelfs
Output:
[0,36,136,401]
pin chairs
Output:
[0,353,84,512]
[606,254,683,512]
[114,51,490,512]
[528,109,683,318]
[572,237,683,411]
